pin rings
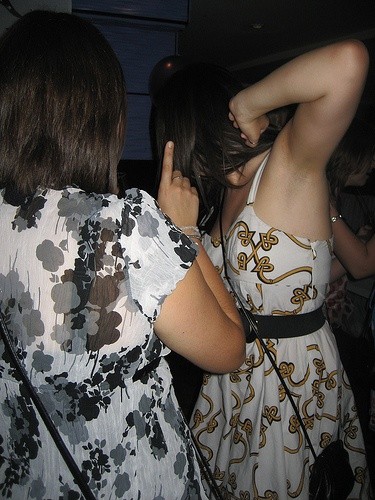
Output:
[172,176,183,183]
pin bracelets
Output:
[181,226,202,241]
[330,215,342,223]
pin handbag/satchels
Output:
[309,439,354,500]
[341,276,375,339]
[335,329,375,389]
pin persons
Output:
[0,9,245,500]
[149,37,371,500]
[325,116,375,338]
